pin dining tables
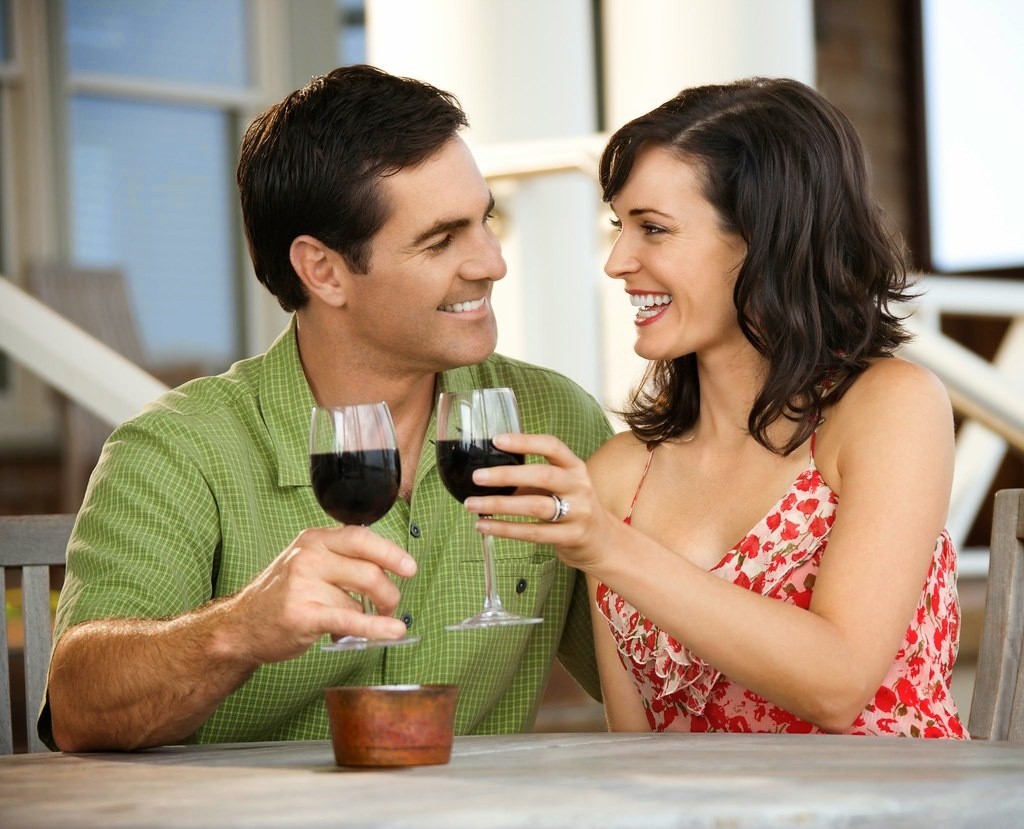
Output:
[0,733,1024,829]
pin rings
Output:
[544,494,567,522]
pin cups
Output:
[324,684,460,767]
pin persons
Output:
[465,75,977,741]
[36,64,619,753]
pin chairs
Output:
[0,514,77,755]
[964,488,1024,741]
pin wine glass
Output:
[435,387,545,630]
[308,401,423,650]
